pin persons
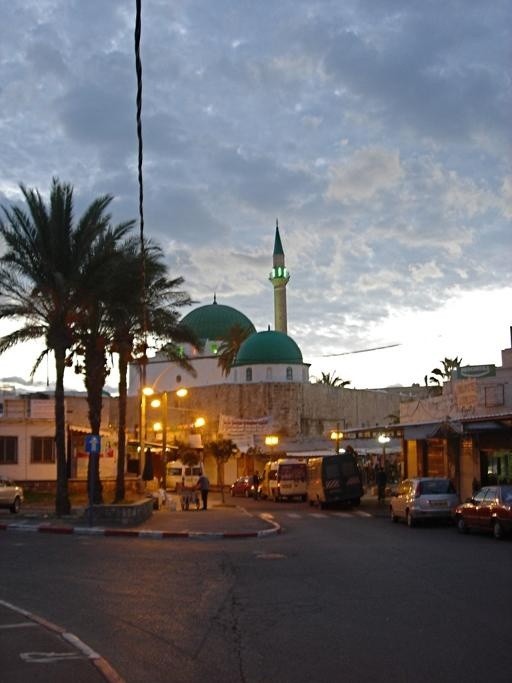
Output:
[253,470,259,502]
[193,471,210,511]
[375,467,387,505]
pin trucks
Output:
[251,457,309,501]
[305,452,364,511]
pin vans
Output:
[159,459,207,494]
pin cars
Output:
[453,484,512,541]
[0,475,26,515]
[388,475,463,529]
[227,474,267,500]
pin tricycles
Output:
[177,484,202,509]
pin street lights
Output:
[143,386,191,496]
[265,434,279,463]
[330,431,345,454]
[378,433,392,474]
